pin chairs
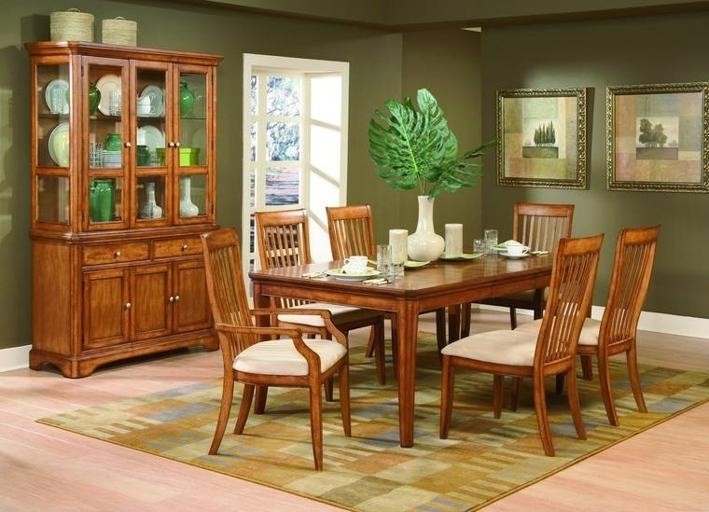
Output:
[439,232,605,457]
[461,202,594,339]
[323,202,452,357]
[254,207,388,402]
[514,223,662,427]
[196,227,354,471]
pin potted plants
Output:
[367,87,498,263]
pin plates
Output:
[95,74,124,116]
[47,122,72,166]
[491,246,531,252]
[142,84,165,118]
[45,78,70,114]
[439,252,483,262]
[324,267,383,282]
[368,258,431,269]
[136,125,165,167]
[100,151,123,169]
[135,112,157,117]
[499,252,530,259]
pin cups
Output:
[390,260,405,279]
[375,243,392,271]
[136,93,156,113]
[484,228,498,254]
[506,244,528,255]
[343,256,369,273]
[472,238,486,254]
[50,80,65,114]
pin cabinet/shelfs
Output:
[23,39,227,378]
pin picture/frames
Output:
[604,83,708,192]
[496,87,589,190]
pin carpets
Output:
[33,328,709,512]
